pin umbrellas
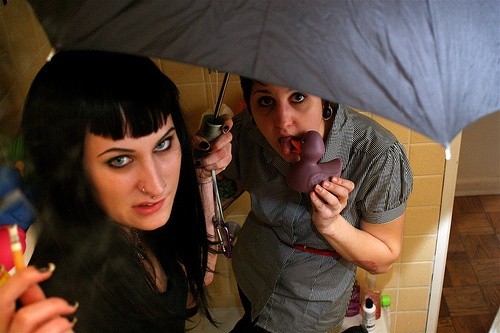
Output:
[26,1,500,158]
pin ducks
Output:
[286,130,342,194]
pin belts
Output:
[294,245,341,257]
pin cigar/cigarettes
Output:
[9,223,25,272]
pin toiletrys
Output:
[362,298,376,332]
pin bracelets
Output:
[197,176,213,184]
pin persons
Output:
[222,76,414,333]
[0,49,233,333]
